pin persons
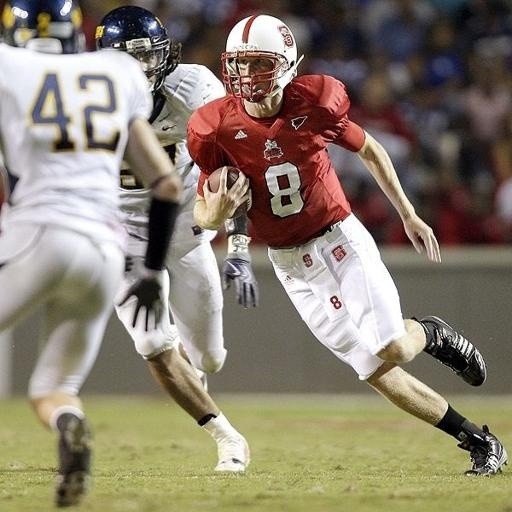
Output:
[95,4,261,474]
[144,1,510,246]
[185,13,509,477]
[0,0,185,507]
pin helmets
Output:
[2,0,86,54]
[94,5,183,94]
[220,15,305,101]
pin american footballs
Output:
[209,166,252,219]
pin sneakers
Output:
[216,435,251,474]
[415,314,485,386]
[55,414,91,509]
[457,422,509,478]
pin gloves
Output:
[116,267,165,331]
[222,235,259,310]
